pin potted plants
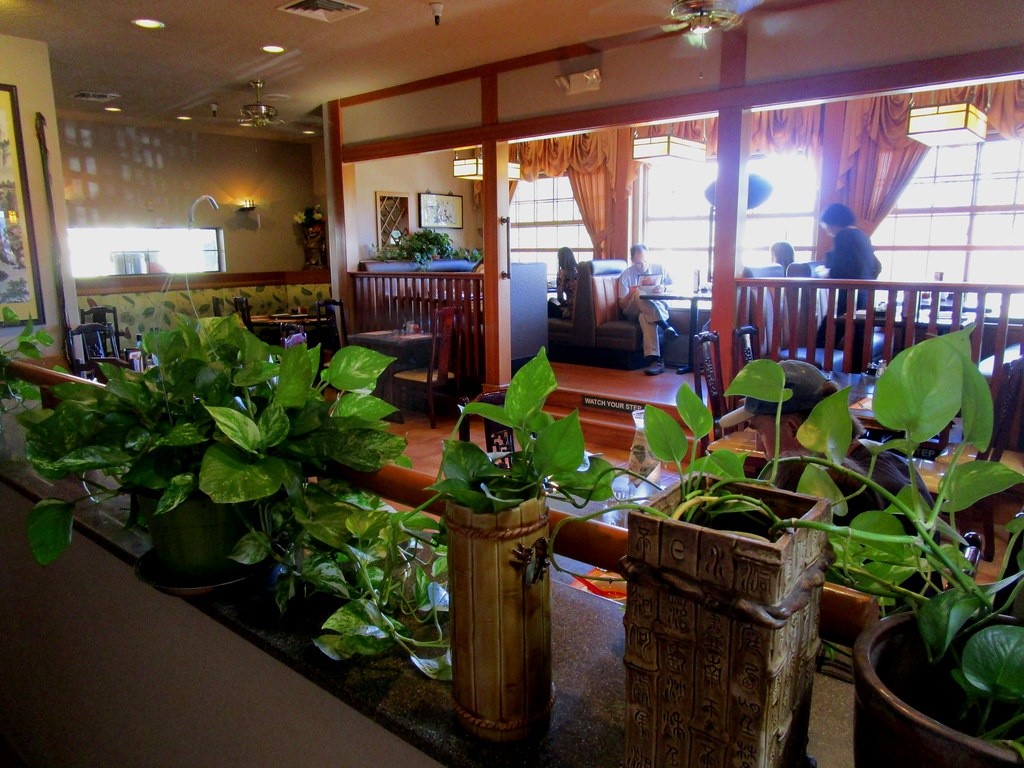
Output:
[0,314,461,687]
[371,229,484,263]
[548,359,1024,768]
[851,599,1024,768]
[434,364,614,740]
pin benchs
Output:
[548,261,646,364]
[363,255,477,323]
[737,256,888,375]
[975,340,1024,421]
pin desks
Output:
[739,374,953,452]
[640,289,713,374]
[348,330,441,425]
[249,315,333,328]
[398,291,482,305]
[547,472,661,529]
[834,311,981,353]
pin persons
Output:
[816,203,882,374]
[548,246,578,321]
[748,359,946,616]
[770,242,794,270]
[618,243,678,376]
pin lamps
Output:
[452,142,521,182]
[906,82,990,149]
[633,119,707,163]
[555,69,601,96]
[239,200,256,211]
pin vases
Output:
[301,232,326,270]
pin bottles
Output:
[875,359,887,376]
[629,409,661,488]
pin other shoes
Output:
[644,358,666,374]
[662,327,679,341]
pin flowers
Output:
[292,203,324,235]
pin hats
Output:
[817,202,854,227]
[743,359,829,416]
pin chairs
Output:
[459,394,536,471]
[901,355,1023,561]
[69,295,465,429]
[943,533,983,592]
[690,330,732,465]
[733,325,762,405]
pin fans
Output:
[172,78,333,136]
[577,0,823,61]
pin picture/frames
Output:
[420,193,464,230]
[0,84,46,327]
